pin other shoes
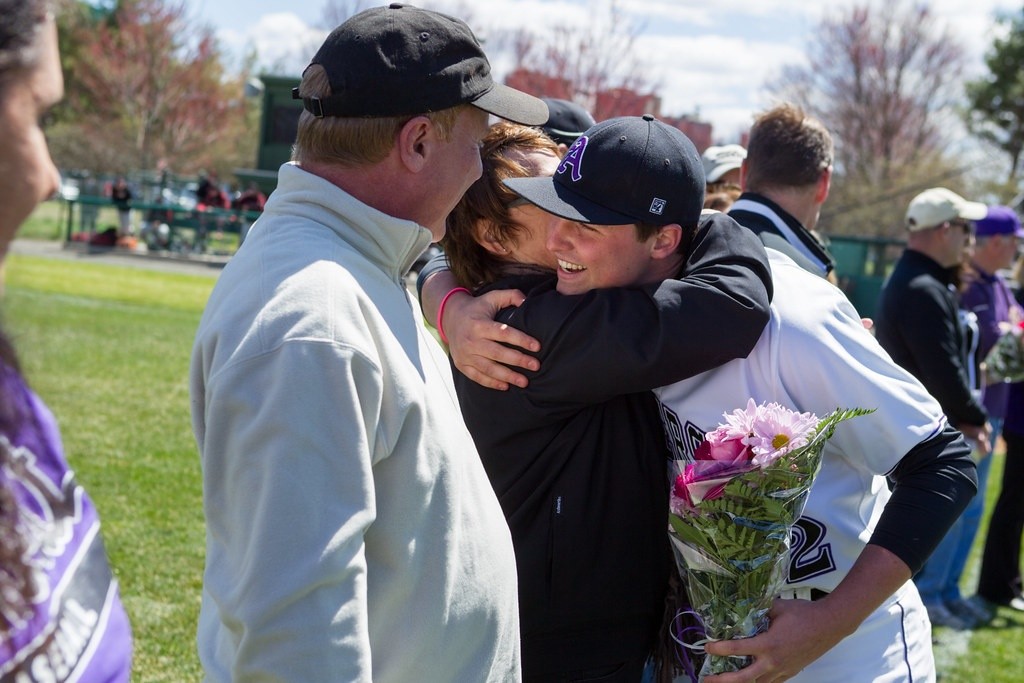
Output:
[1010,581,1024,611]
[922,600,990,630]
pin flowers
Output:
[666,397,881,683]
[981,305,1023,385]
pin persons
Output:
[874,187,986,644]
[726,106,872,331]
[185,3,552,683]
[925,207,1024,627]
[188,180,231,256]
[977,252,1024,608]
[111,175,132,236]
[698,144,747,211]
[0,0,133,683]
[140,198,174,250]
[234,190,267,247]
[438,124,776,682]
[415,112,979,682]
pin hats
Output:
[974,205,1024,239]
[701,144,748,183]
[538,97,598,146]
[499,114,705,227]
[292,1,550,127]
[905,186,988,232]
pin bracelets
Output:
[438,285,472,344]
